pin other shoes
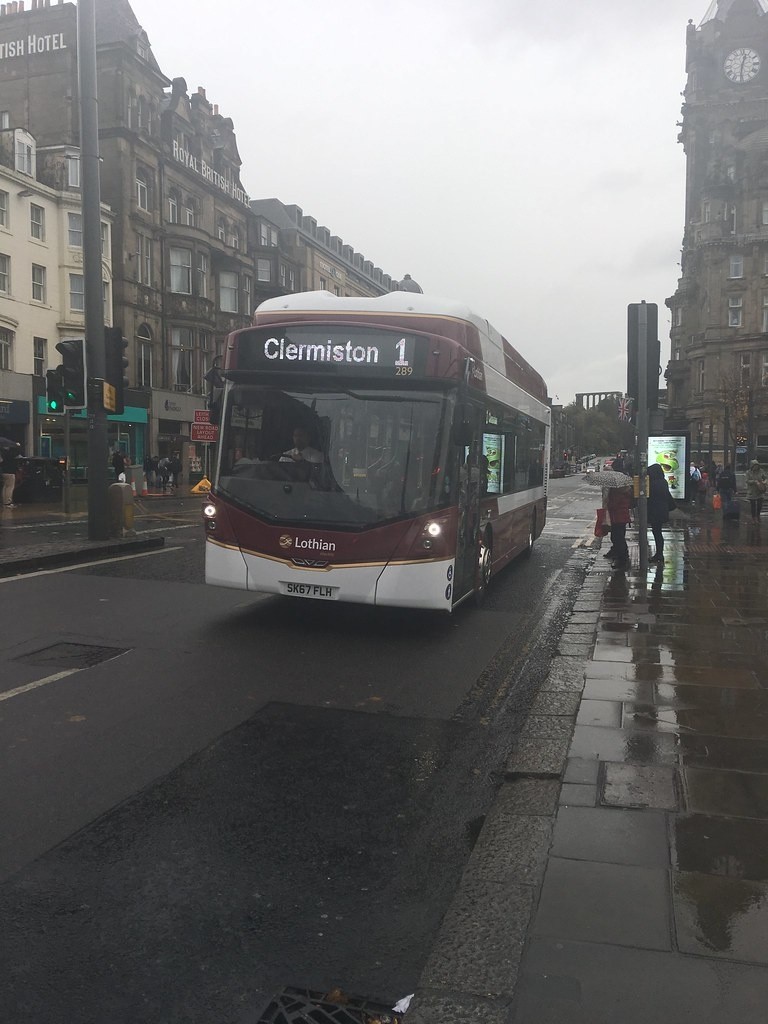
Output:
[3,504,16,510]
[603,546,616,558]
[649,552,664,563]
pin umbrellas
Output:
[582,470,634,489]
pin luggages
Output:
[726,494,739,521]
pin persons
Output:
[2,442,22,508]
[647,464,676,563]
[278,426,324,464]
[112,449,124,482]
[602,458,631,568]
[149,455,183,491]
[744,459,766,523]
[690,460,738,520]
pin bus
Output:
[203,288,553,612]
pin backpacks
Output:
[692,467,699,482]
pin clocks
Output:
[724,46,761,83]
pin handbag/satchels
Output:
[594,509,608,537]
[713,494,721,510]
[667,491,676,511]
[118,472,126,483]
[757,480,767,492]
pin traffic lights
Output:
[45,369,66,415]
[54,339,87,409]
[105,326,129,416]
